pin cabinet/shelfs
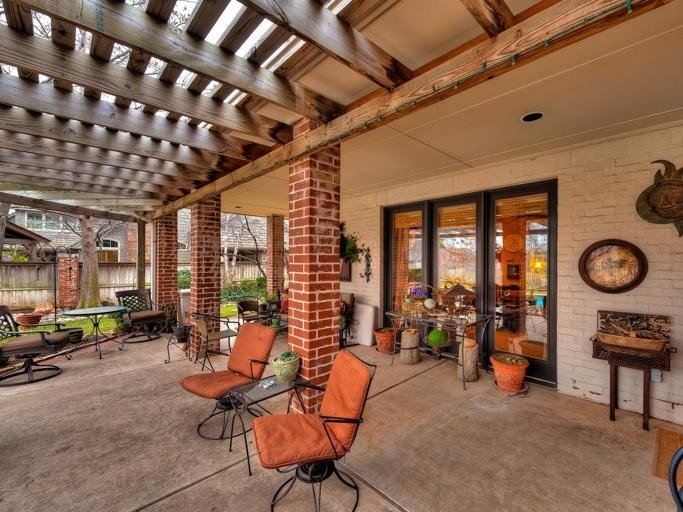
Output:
[588,337,677,431]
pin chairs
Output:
[251,348,377,512]
[116,289,166,343]
[180,322,277,440]
[236,295,264,331]
[0,304,70,387]
[190,312,240,372]
[338,292,359,348]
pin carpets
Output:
[649,426,683,486]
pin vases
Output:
[171,323,191,343]
[17,313,42,329]
[52,327,83,343]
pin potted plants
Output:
[490,353,530,396]
[518,340,547,360]
[273,350,300,384]
[374,326,399,354]
[271,315,281,328]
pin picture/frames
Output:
[578,239,648,294]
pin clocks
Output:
[503,232,524,253]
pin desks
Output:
[385,310,493,390]
[62,306,127,360]
[228,373,307,476]
